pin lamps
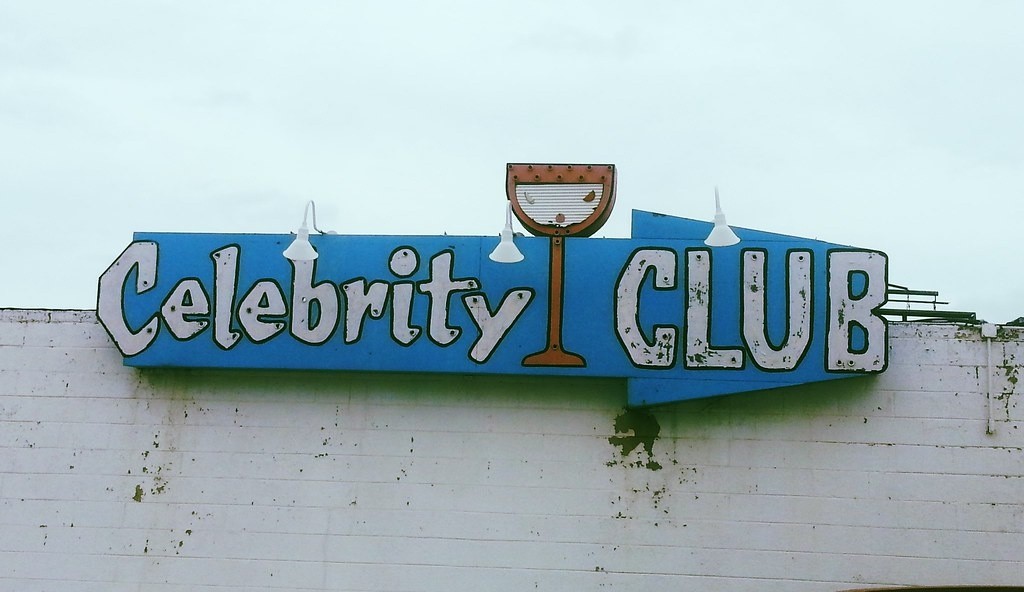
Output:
[283,201,337,261]
[704,187,740,246]
[489,200,524,263]
